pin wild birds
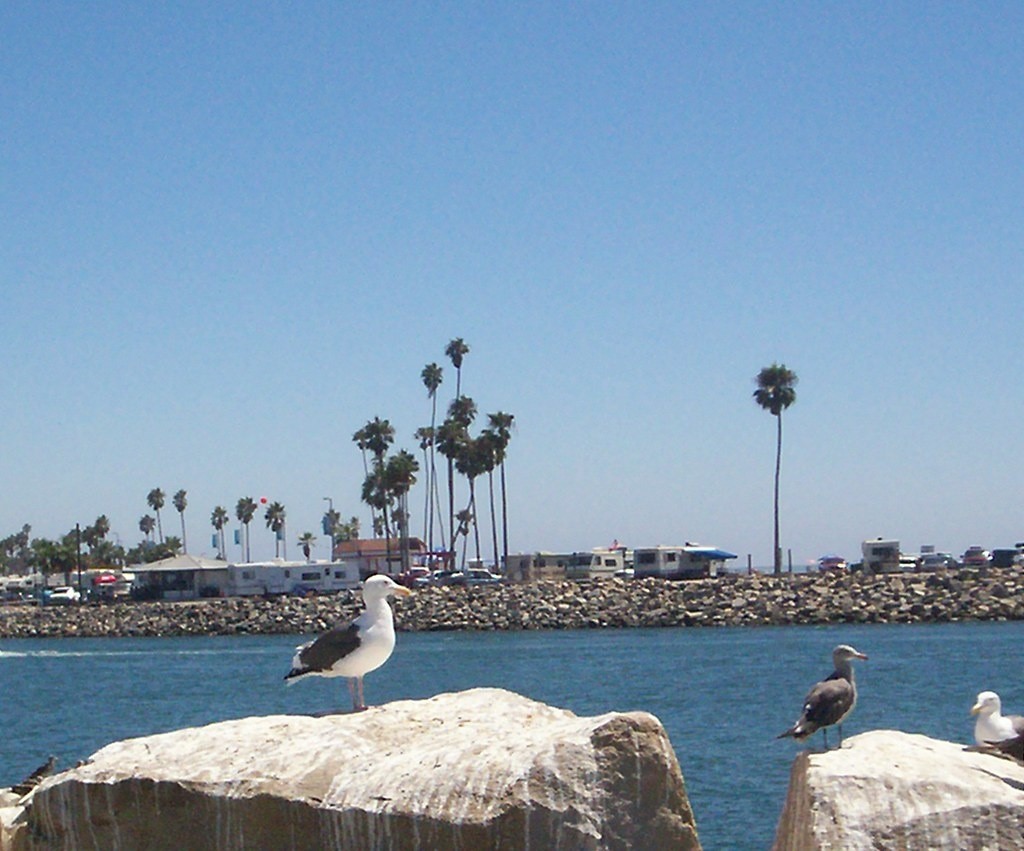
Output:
[281,573,414,710]
[770,644,869,755]
[970,691,1024,762]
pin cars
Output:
[386,567,431,587]
[455,568,503,584]
[991,549,1023,567]
[808,554,849,573]
[413,569,461,594]
[960,548,992,565]
[50,586,80,605]
[919,555,958,572]
[104,584,132,603]
[899,556,920,573]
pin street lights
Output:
[323,497,335,563]
